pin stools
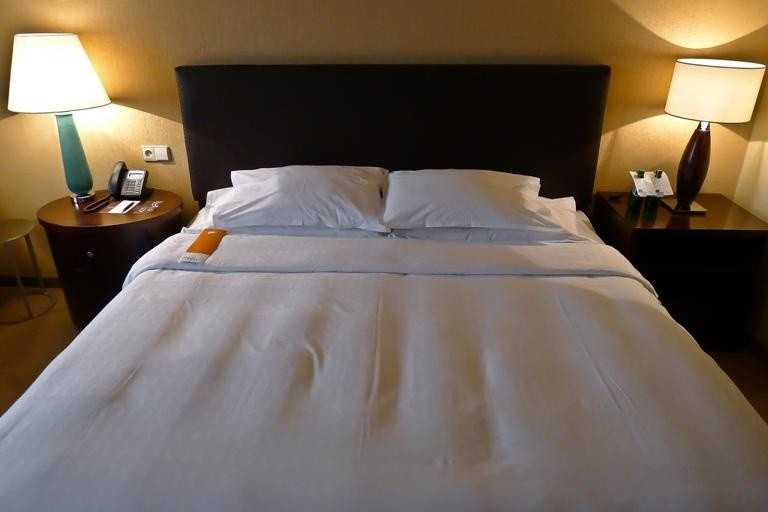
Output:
[0,219,56,325]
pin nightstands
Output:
[36,188,183,334]
[592,191,768,354]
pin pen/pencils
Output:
[122,202,134,212]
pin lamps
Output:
[659,58,766,215]
[7,32,113,204]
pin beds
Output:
[0,62,768,512]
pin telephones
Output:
[108,161,149,199]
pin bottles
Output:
[624,168,663,227]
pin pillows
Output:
[212,165,393,233]
[387,195,577,243]
[379,169,541,229]
[188,187,383,238]
